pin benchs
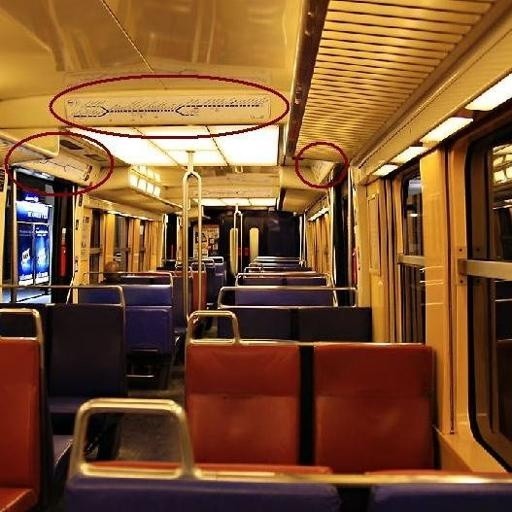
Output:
[0,256,512,512]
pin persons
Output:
[97,262,130,285]
